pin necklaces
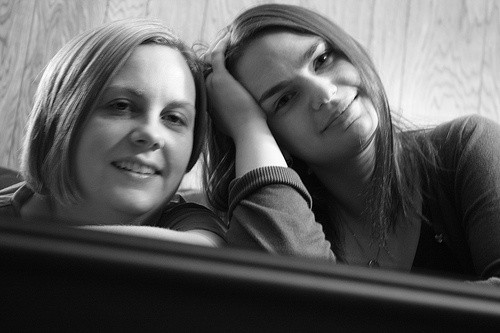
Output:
[335,204,383,270]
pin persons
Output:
[202,4,500,288]
[0,15,227,247]
